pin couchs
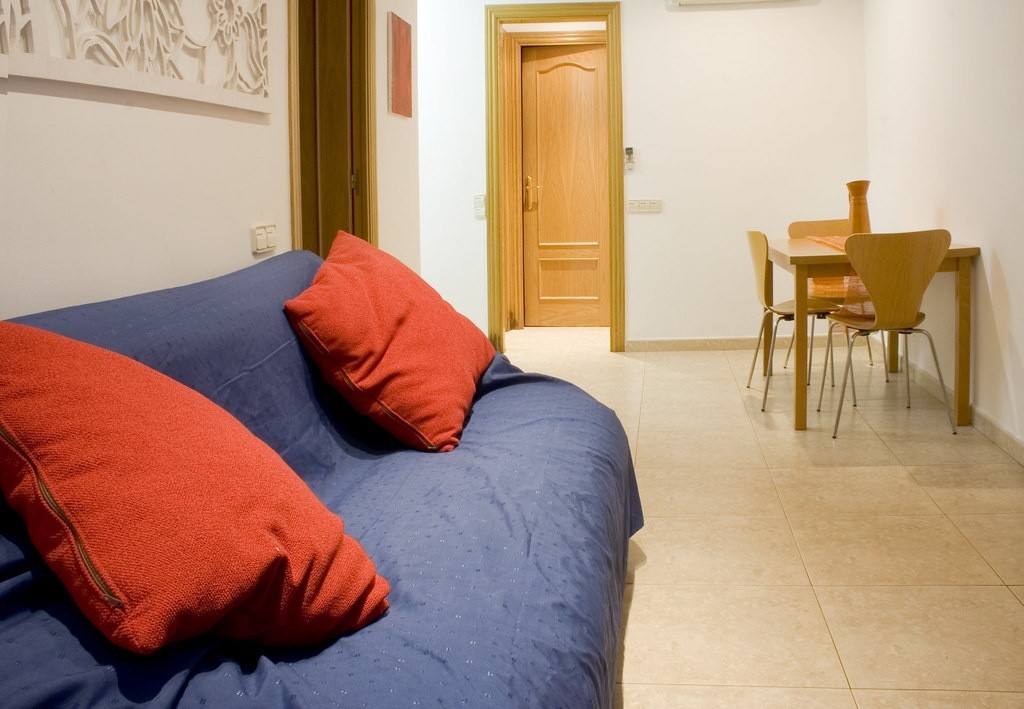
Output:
[0,247,644,709]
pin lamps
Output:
[624,148,634,170]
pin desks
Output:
[767,234,982,430]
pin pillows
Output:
[283,229,498,455]
[0,319,392,654]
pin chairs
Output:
[747,218,981,440]
